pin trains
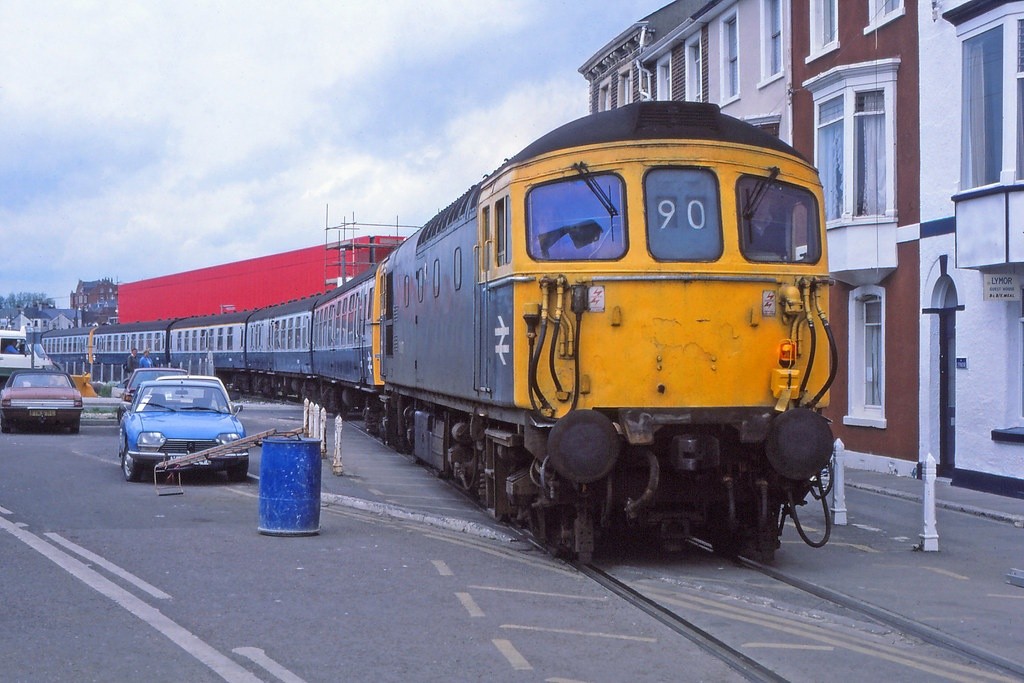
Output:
[41,101,840,564]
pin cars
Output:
[115,366,190,422]
[118,380,252,484]
[0,367,86,435]
[149,373,230,401]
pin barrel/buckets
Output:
[259,439,322,537]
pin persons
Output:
[123,347,137,391]
[2,339,22,354]
[139,350,155,369]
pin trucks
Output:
[0,330,54,375]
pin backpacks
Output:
[123,355,133,373]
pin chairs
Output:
[194,398,212,408]
[146,394,166,405]
